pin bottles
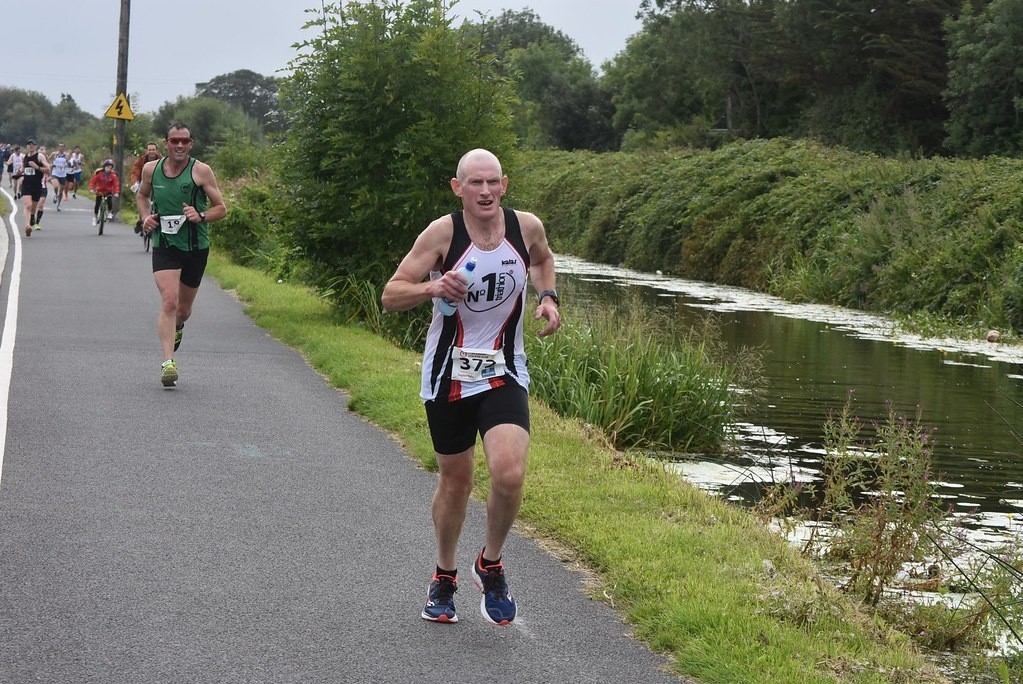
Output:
[438,257,478,316]
[132,182,139,191]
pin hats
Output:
[26,139,36,144]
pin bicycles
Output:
[131,185,153,252]
[91,192,117,236]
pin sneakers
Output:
[472,546,517,625]
[160,359,179,387]
[421,570,459,623]
[174,322,185,352]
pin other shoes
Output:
[72,194,76,199]
[26,226,31,236]
[106,213,112,219]
[18,193,21,199]
[30,214,35,225]
[57,204,61,211]
[14,195,17,200]
[135,220,141,233]
[35,223,40,230]
[53,194,58,203]
[64,195,68,200]
[92,216,98,226]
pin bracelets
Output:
[38,167,40,170]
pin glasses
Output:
[165,137,192,145]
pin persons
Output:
[131,142,163,186]
[90,159,120,226]
[34,173,48,230]
[381,148,561,626]
[16,139,50,237]
[0,143,25,200]
[39,142,85,211]
[137,123,227,387]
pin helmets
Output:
[103,159,114,167]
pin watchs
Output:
[538,290,560,307]
[199,212,205,223]
